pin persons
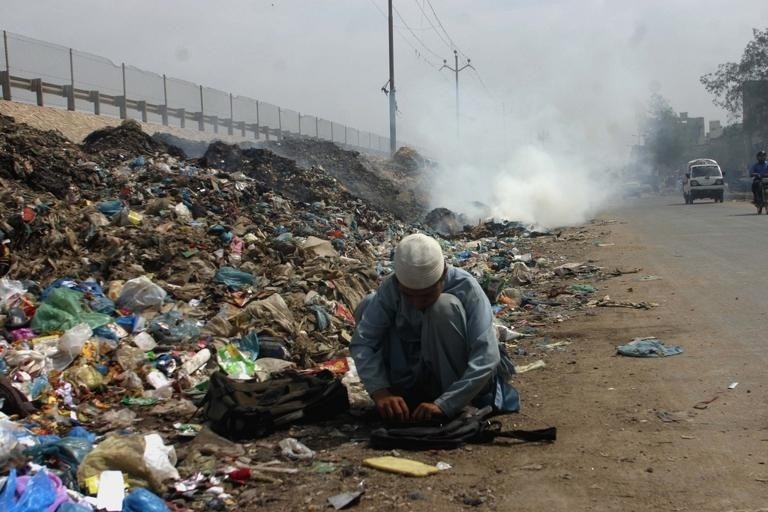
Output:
[748,150,768,208]
[348,232,520,425]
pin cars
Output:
[623,182,641,199]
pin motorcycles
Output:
[750,172,767,216]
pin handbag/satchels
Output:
[371,403,502,451]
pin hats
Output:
[393,233,445,289]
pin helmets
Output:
[757,150,767,162]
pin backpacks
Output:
[205,368,351,443]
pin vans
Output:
[682,158,725,204]
